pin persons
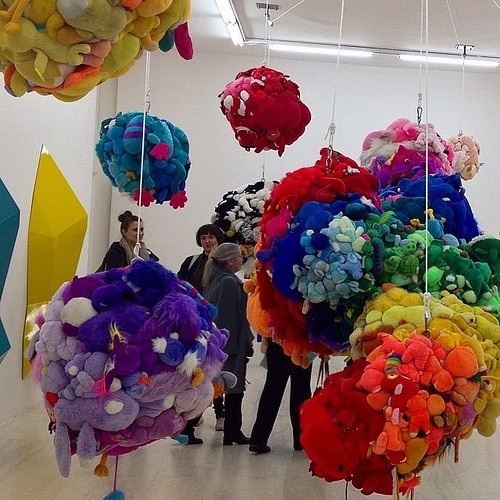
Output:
[94,209,160,276]
[179,243,255,446]
[249,334,332,455]
[176,224,227,431]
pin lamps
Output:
[216,0,246,47]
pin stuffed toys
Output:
[218,66,312,157]
[27,259,238,500]
[211,117,500,500]
[94,111,191,209]
[0,0,194,102]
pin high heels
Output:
[171,425,203,445]
[223,430,251,445]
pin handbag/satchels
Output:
[313,359,330,396]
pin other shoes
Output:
[215,418,225,431]
[248,444,271,453]
[197,417,204,426]
[293,443,303,451]
